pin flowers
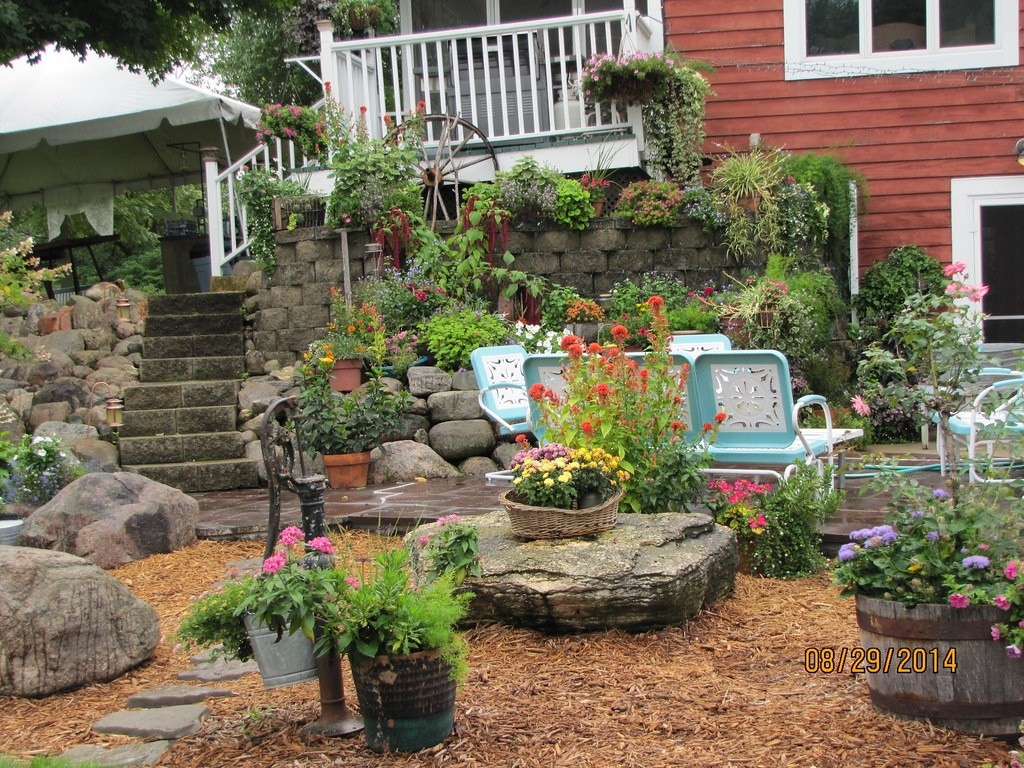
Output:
[176,51,1024,695]
[0,431,87,513]
[503,432,631,506]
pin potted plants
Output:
[703,136,792,211]
[282,370,414,491]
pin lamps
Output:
[85,381,124,438]
[103,283,130,353]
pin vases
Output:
[1,518,24,549]
[732,543,766,576]
[376,356,425,380]
[589,200,605,217]
[722,316,748,347]
[854,591,1023,736]
[240,603,319,689]
[328,360,362,391]
[815,408,848,425]
[567,322,601,344]
[577,488,605,511]
[349,636,457,751]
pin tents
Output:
[0,41,298,258]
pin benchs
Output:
[520,350,864,510]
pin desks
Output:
[30,233,122,298]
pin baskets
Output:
[498,483,623,539]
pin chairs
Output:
[925,367,1024,484]
[669,332,730,359]
[470,345,536,480]
